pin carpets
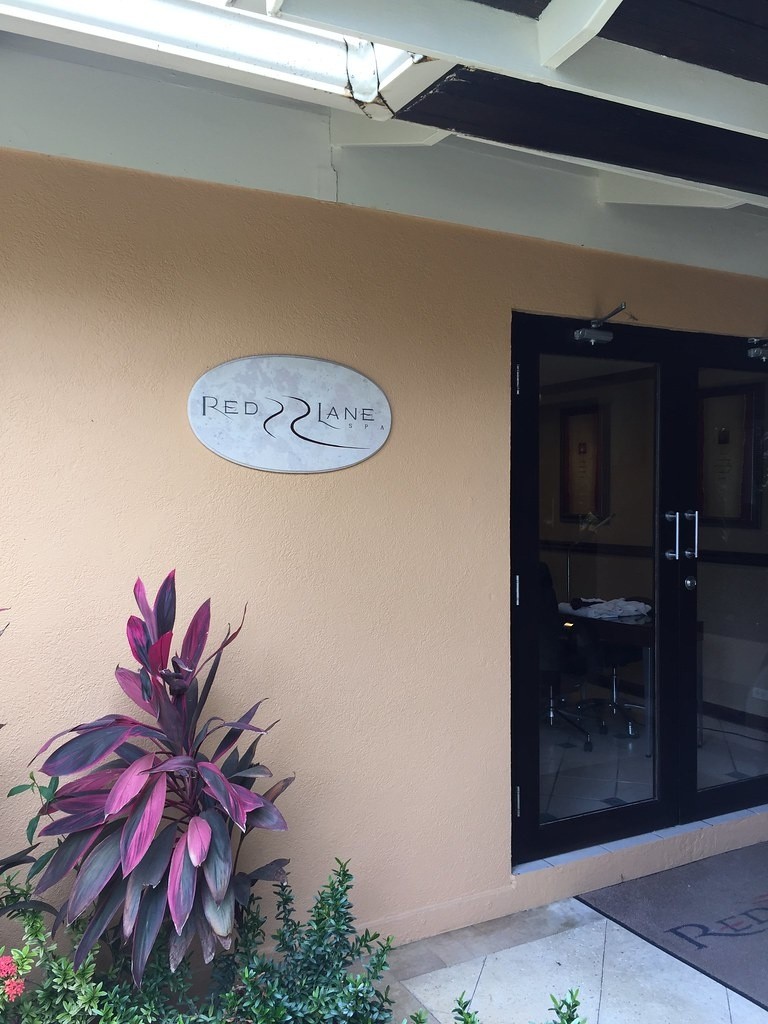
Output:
[573,841,768,1012]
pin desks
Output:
[558,595,704,756]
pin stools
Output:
[577,643,647,741]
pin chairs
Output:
[535,560,609,754]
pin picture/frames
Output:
[698,383,762,529]
[560,404,611,525]
